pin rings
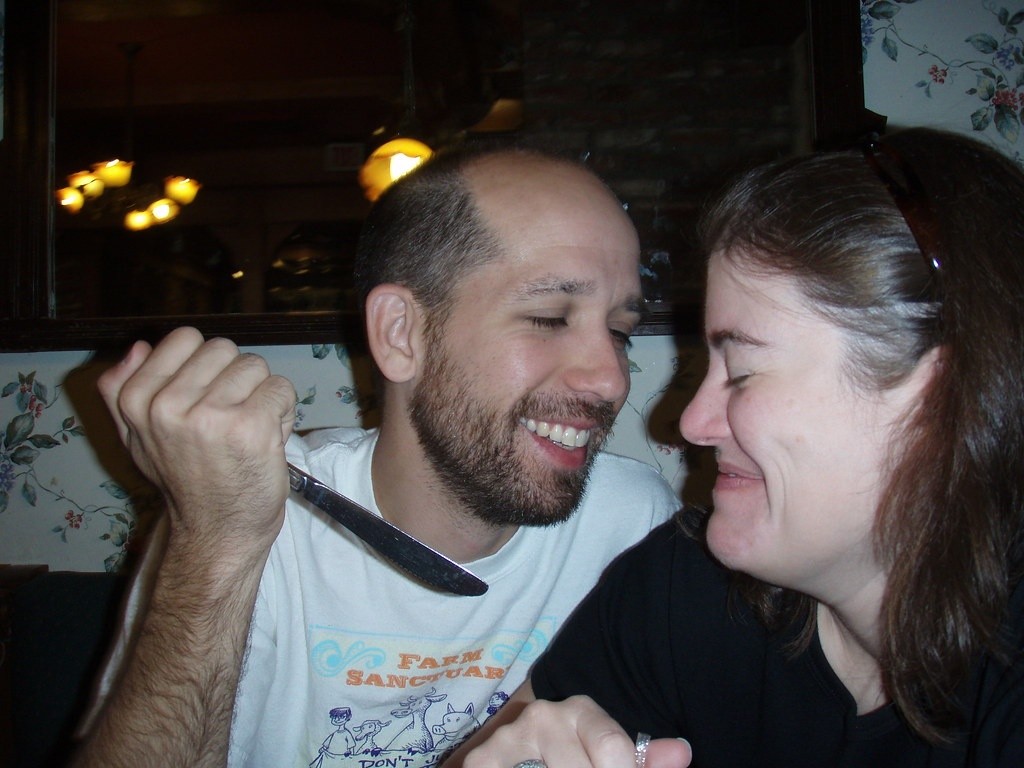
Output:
[515,760,547,768]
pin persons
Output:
[464,127,1024,768]
[84,149,687,768]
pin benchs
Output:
[0,329,718,768]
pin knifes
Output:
[287,460,489,597]
